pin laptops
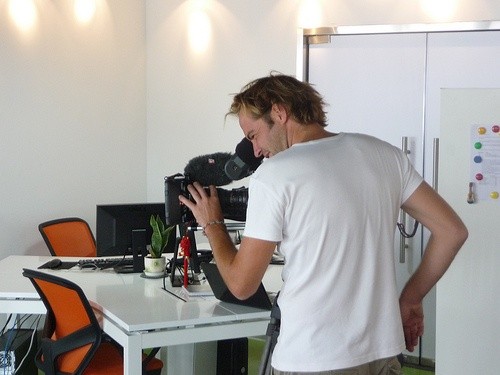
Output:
[201,262,277,311]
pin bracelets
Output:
[203,220,227,238]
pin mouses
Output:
[47,259,61,269]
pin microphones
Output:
[183,152,235,187]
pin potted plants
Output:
[141,216,175,278]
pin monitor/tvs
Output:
[97,203,176,273]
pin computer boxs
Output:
[0,328,38,375]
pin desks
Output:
[0,242,288,375]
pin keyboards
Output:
[78,257,134,269]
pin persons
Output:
[178,69,471,375]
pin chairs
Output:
[37,217,97,258]
[21,267,164,375]
